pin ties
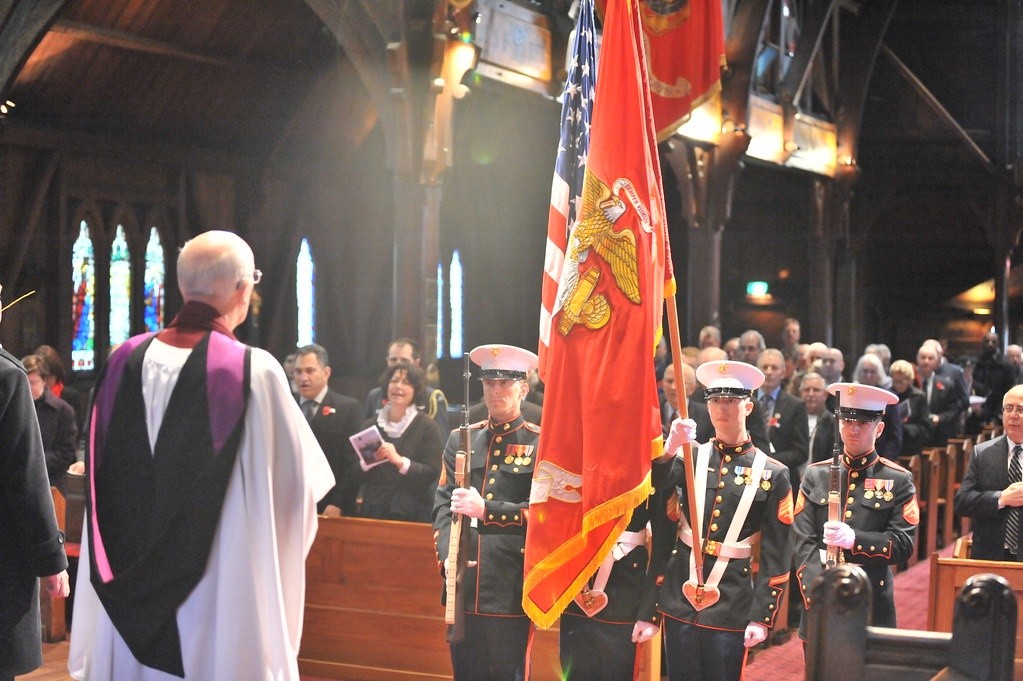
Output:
[922,378,928,404]
[1004,445,1023,555]
[666,411,677,438]
[304,399,318,422]
[760,394,772,444]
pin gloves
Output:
[630,620,661,643]
[823,521,856,550]
[448,486,486,518]
[665,416,697,456]
[443,555,478,589]
[744,619,769,647]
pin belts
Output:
[817,548,865,567]
[676,514,753,559]
[614,530,648,547]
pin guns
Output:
[827,388,845,572]
[451,350,472,594]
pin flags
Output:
[523,0,726,629]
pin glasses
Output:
[1002,404,1023,416]
[235,270,263,290]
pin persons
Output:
[20,319,1023,523]
[559,485,683,681]
[0,286,70,681]
[794,382,919,666]
[954,384,1023,562]
[430,345,540,681]
[68,229,335,681]
[650,360,793,681]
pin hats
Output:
[469,343,540,381]
[826,382,900,421]
[695,360,766,400]
